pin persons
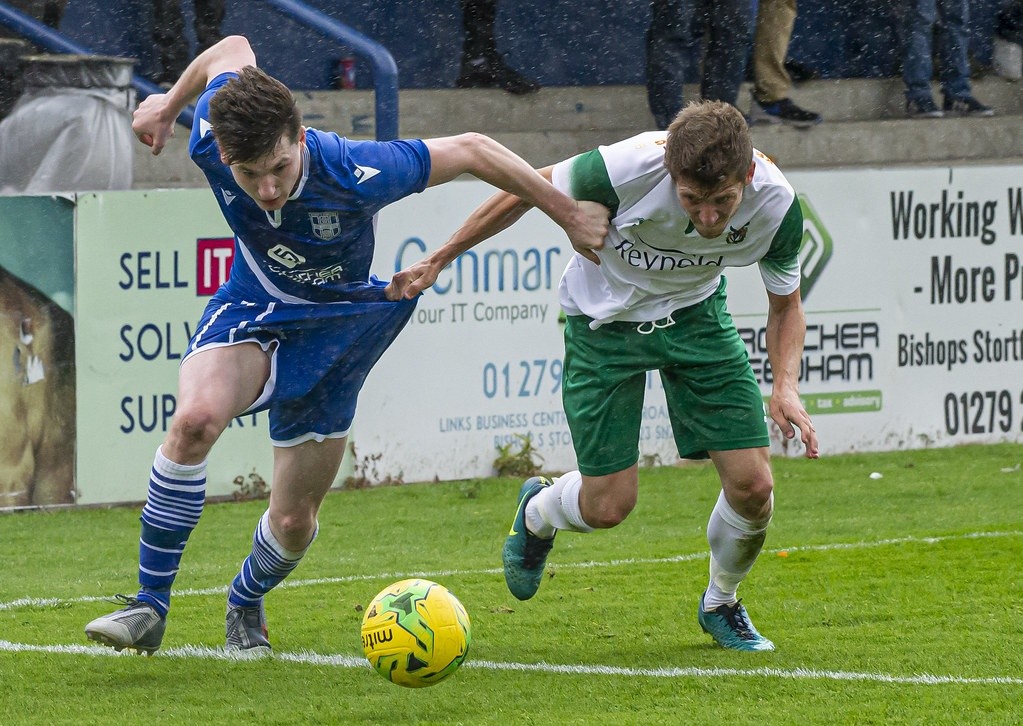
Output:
[900,0,995,118]
[754,0,821,129]
[83,36,611,656]
[385,102,818,655]
[152,0,228,92]
[645,0,759,130]
[459,0,536,91]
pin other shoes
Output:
[454,50,541,95]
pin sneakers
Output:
[84,594,165,659]
[698,589,776,656]
[904,92,944,118]
[941,95,996,118]
[747,93,821,128]
[225,579,273,657]
[502,476,559,602]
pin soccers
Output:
[360,577,472,689]
